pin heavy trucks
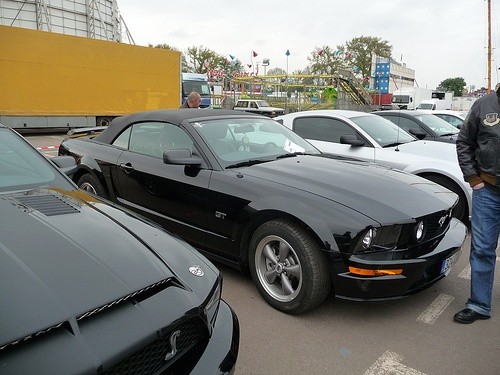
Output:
[0,26,183,131]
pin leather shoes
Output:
[453,308,490,322]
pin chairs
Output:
[143,125,234,158]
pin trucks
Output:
[182,72,214,108]
[391,86,430,110]
[416,98,445,111]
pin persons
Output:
[178,91,201,109]
[453,84,500,324]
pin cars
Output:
[272,109,473,228]
[368,111,461,143]
[429,111,466,129]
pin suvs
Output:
[234,99,285,118]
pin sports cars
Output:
[0,126,240,375]
[56,107,468,314]
